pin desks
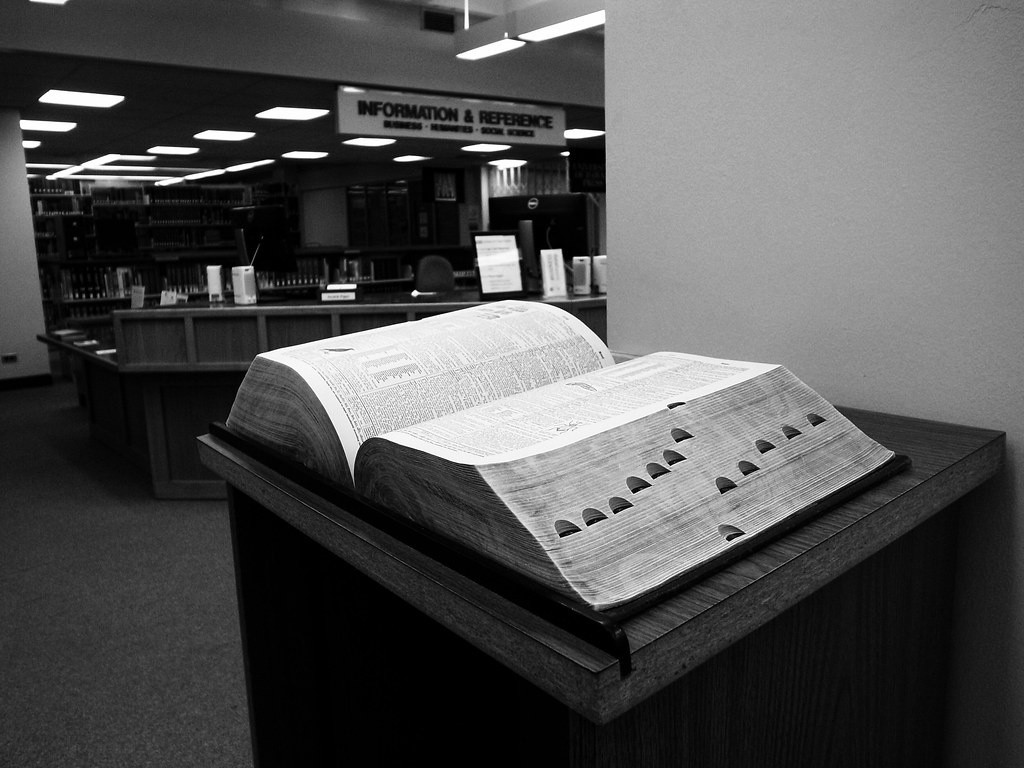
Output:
[196,367,1024,768]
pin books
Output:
[225,298,912,629]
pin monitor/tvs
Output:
[489,193,596,287]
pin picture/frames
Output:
[469,230,530,301]
